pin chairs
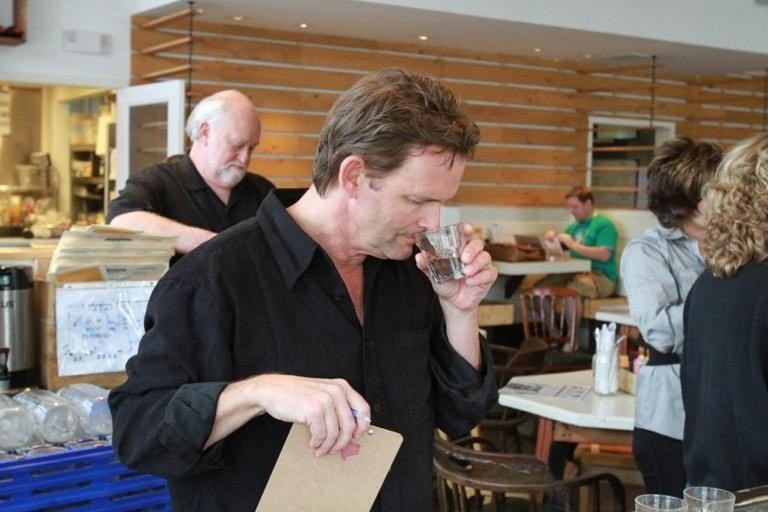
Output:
[514,281,592,368]
[425,427,630,510]
[477,334,553,450]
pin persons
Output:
[106,90,275,256]
[681,132,768,496]
[533,185,618,354]
[620,138,724,495]
[107,67,498,512]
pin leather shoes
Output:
[561,340,581,354]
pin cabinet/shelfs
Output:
[68,141,112,227]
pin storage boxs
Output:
[14,162,48,189]
[33,269,163,393]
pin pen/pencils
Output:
[351,409,372,423]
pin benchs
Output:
[519,214,649,315]
[472,300,513,330]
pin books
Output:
[49,221,180,280]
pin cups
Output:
[590,353,619,397]
[634,494,688,512]
[414,222,464,283]
[684,487,735,511]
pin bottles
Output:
[474,227,482,240]
[0,194,20,225]
[0,382,115,464]
